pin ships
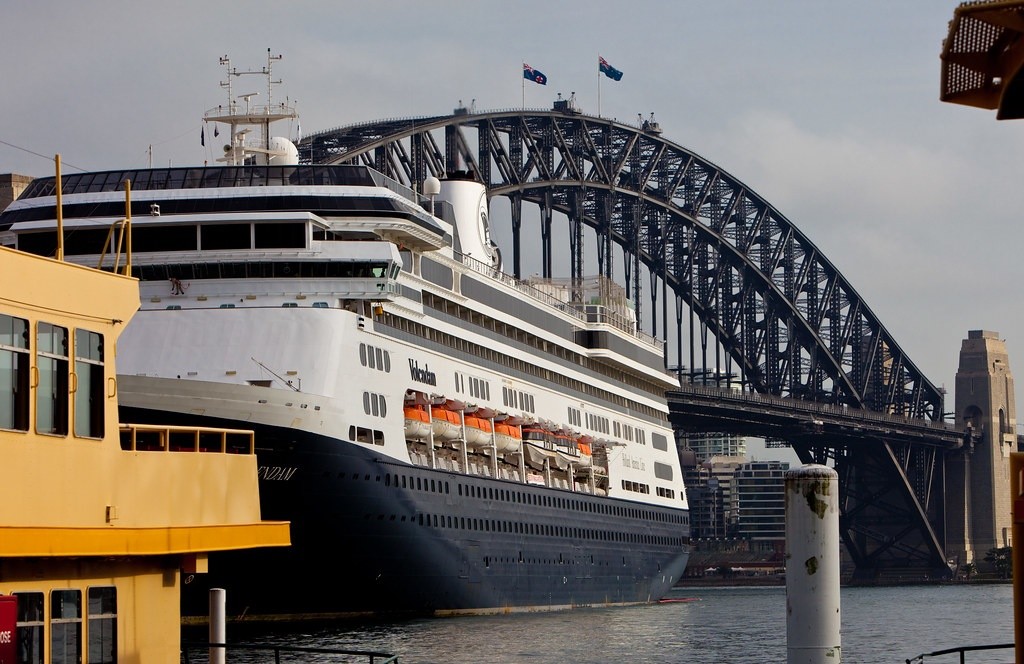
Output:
[0,45,694,626]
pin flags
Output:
[524,63,548,86]
[213,123,220,138]
[296,118,302,145]
[200,125,205,146]
[599,56,624,82]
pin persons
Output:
[169,277,185,295]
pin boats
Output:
[494,423,522,466]
[463,413,493,458]
[554,434,581,472]
[403,403,430,443]
[432,405,462,453]
[523,428,558,472]
[574,442,592,477]
